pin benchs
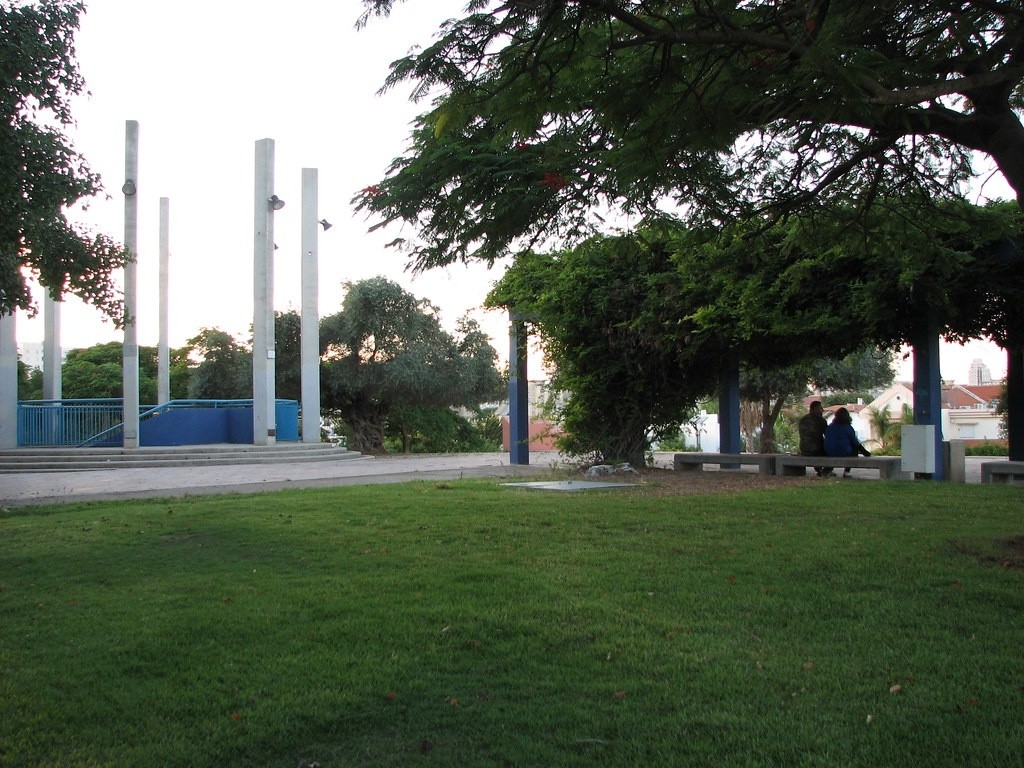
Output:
[774,455,902,480]
[981,461,1024,485]
[673,453,781,475]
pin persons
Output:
[824,408,870,479]
[799,401,833,478]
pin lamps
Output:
[122,179,136,195]
[318,219,333,231]
[268,195,285,210]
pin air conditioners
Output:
[974,404,983,409]
[960,406,971,409]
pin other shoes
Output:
[843,472,852,477]
[824,472,837,476]
